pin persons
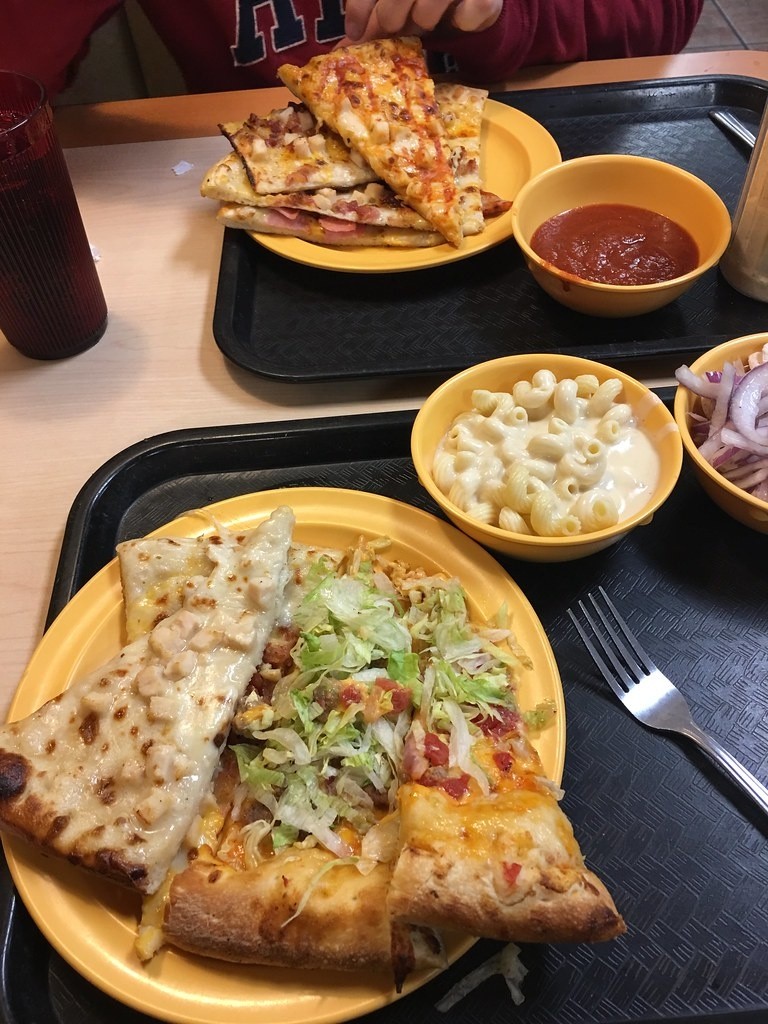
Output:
[0,0,708,110]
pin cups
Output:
[0,68,110,363]
[717,97,768,301]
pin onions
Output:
[675,343,768,504]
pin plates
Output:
[1,486,569,1023]
[245,98,564,275]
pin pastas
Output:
[434,370,633,538]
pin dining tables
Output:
[0,45,768,1024]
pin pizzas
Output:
[199,36,513,247]
[0,501,627,979]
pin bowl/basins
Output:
[409,354,684,564]
[509,152,734,319]
[675,332,768,537]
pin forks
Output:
[563,585,768,820]
